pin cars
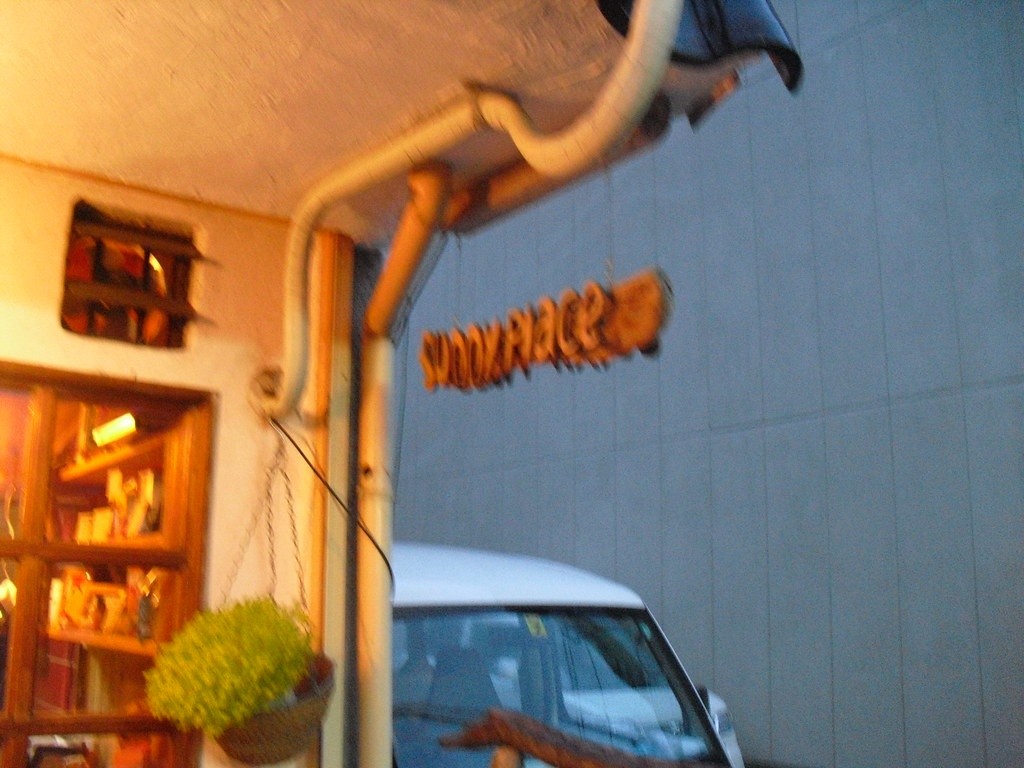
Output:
[453,610,743,766]
[388,543,745,768]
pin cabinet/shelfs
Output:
[3,362,216,655]
